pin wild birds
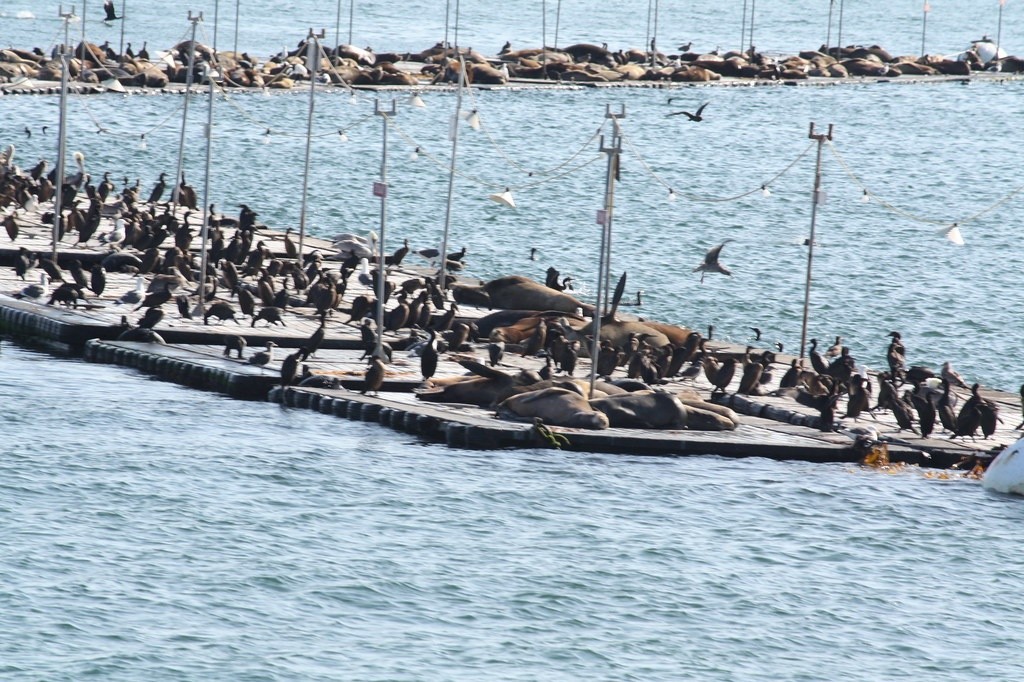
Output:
[0,1,1024,444]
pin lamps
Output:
[936,223,964,245]
[63,15,80,23]
[200,67,219,78]
[155,51,176,70]
[490,187,516,209]
[405,92,425,108]
[462,109,480,129]
[102,77,126,93]
